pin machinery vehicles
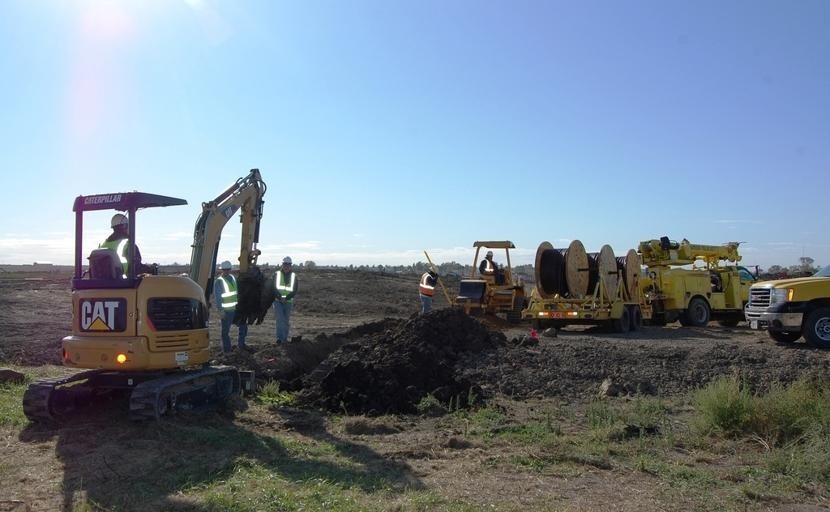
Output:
[454,240,528,325]
[528,235,763,332]
[23,167,282,423]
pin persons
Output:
[100,213,152,279]
[270,255,298,343]
[419,265,439,312]
[214,260,249,354]
[479,251,503,284]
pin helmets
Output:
[486,251,493,256]
[219,261,231,270]
[111,214,128,227]
[429,266,437,275]
[282,256,292,263]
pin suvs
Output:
[742,264,830,347]
